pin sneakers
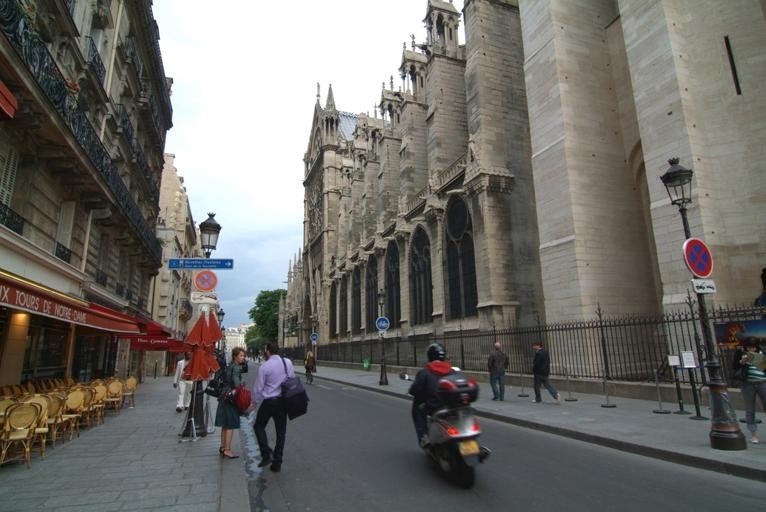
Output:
[258,452,282,472]
[532,399,540,403]
[750,436,760,444]
[175,407,189,413]
[493,394,505,402]
[556,392,561,402]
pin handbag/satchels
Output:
[205,377,224,399]
[230,386,251,412]
[734,363,750,381]
[280,361,309,419]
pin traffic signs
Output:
[167,259,232,269]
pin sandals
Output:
[219,447,239,459]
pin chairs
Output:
[0,374,138,470]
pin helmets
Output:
[427,342,446,362]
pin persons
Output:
[249,343,297,472]
[407,342,456,448]
[531,342,561,403]
[487,341,509,401]
[303,351,316,384]
[731,335,766,444]
[214,347,249,458]
[173,352,193,412]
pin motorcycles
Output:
[394,367,490,491]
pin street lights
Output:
[375,288,389,385]
[177,208,223,440]
[657,153,749,453]
[310,312,318,373]
